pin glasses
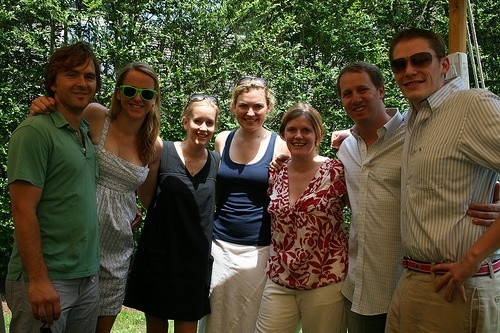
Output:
[236,77,269,93]
[184,94,219,110]
[390,51,443,74]
[119,84,157,101]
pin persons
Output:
[268,60,499,333]
[208,80,291,332]
[254,101,351,333]
[143,90,223,332]
[6,42,143,333]
[330,28,499,333]
[27,61,163,333]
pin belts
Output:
[402,255,500,275]
